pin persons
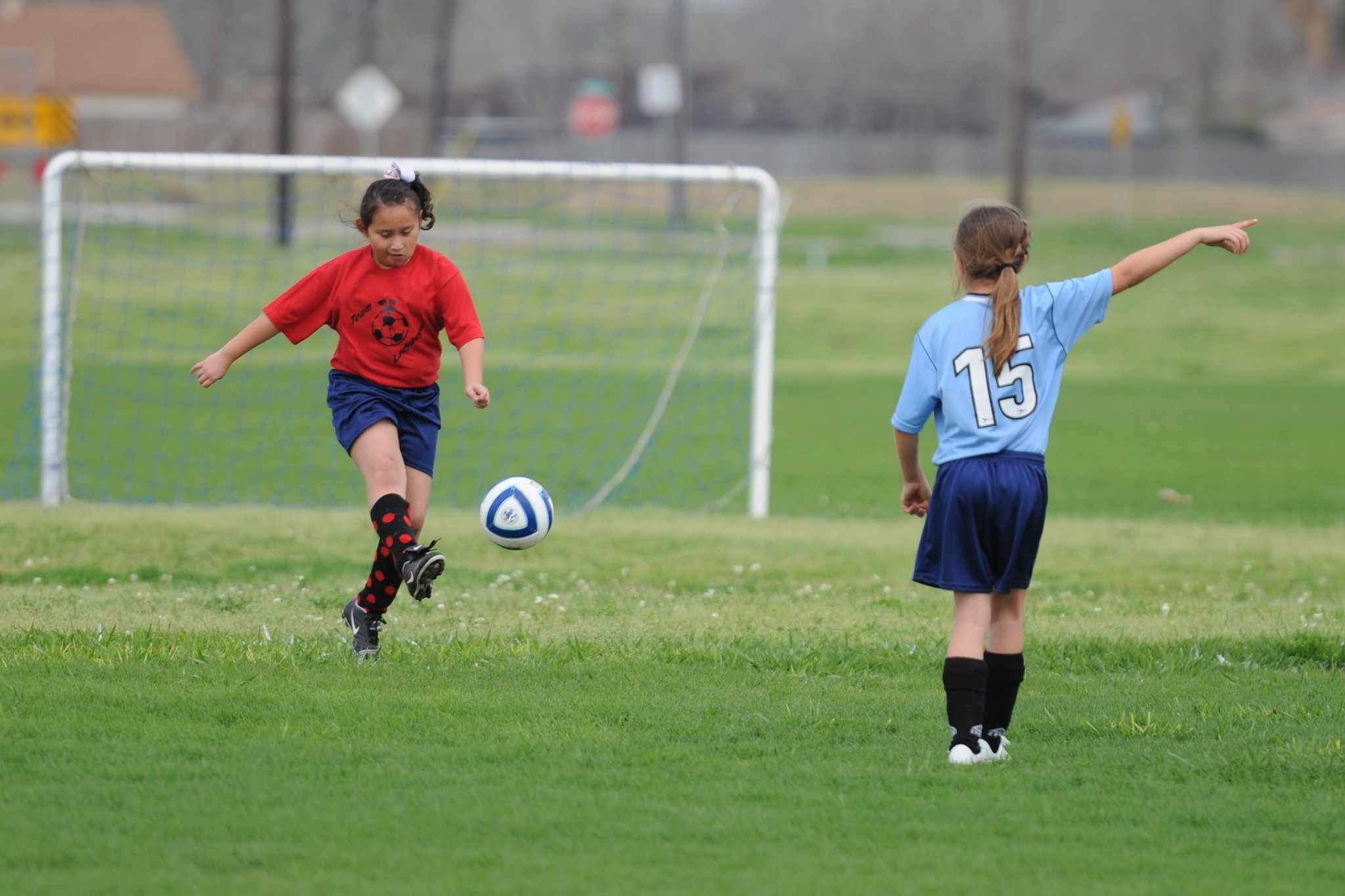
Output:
[889,207,1257,762]
[188,164,491,659]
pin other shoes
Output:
[977,735,1010,764]
[948,740,982,766]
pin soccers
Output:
[482,475,552,549]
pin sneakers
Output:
[342,595,387,659]
[402,544,445,600]
